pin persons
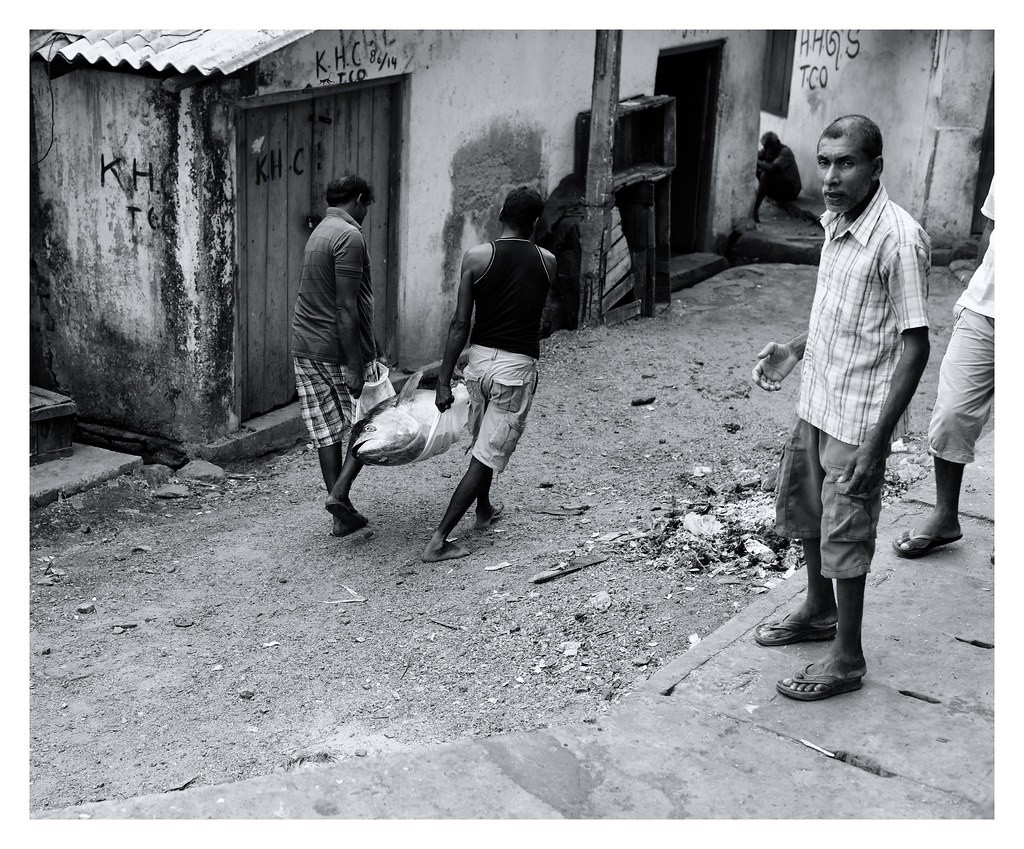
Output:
[893,165,995,558]
[754,130,802,222]
[752,115,931,702]
[288,174,388,538]
[421,186,556,562]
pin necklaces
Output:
[836,215,853,238]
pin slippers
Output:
[891,522,963,559]
[323,501,369,530]
[776,662,863,701]
[755,612,839,646]
[328,526,362,537]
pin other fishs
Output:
[348,364,473,465]
